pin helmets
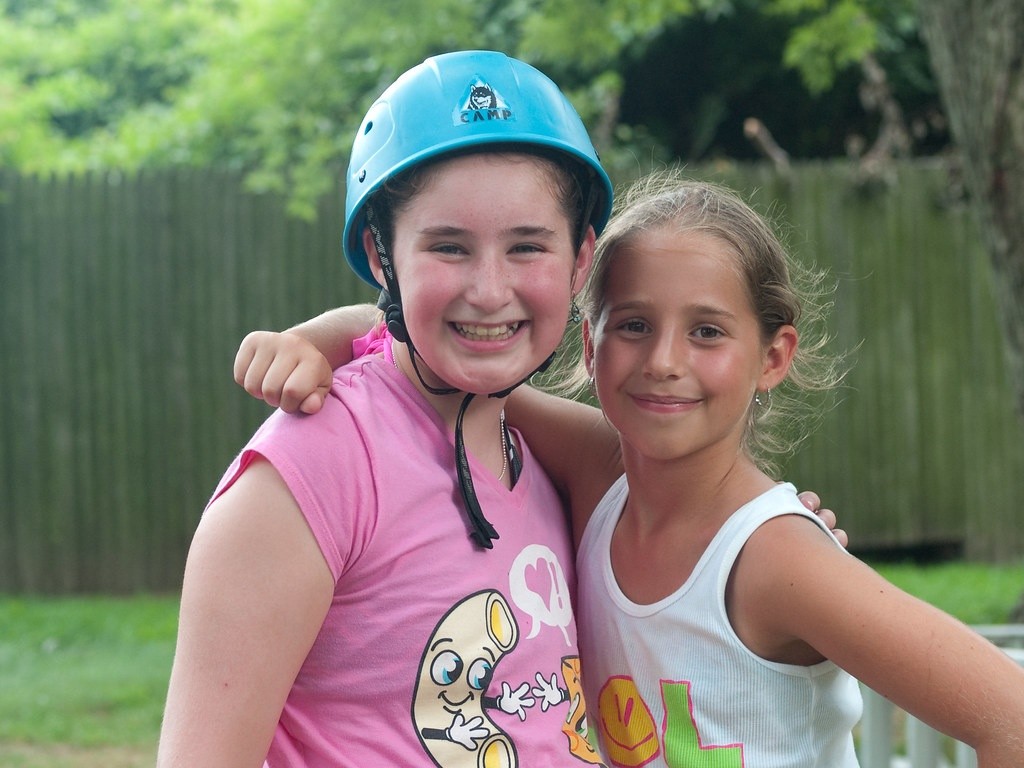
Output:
[343,50,614,294]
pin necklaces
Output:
[494,411,507,482]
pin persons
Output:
[234,167,1023,768]
[157,49,848,768]
